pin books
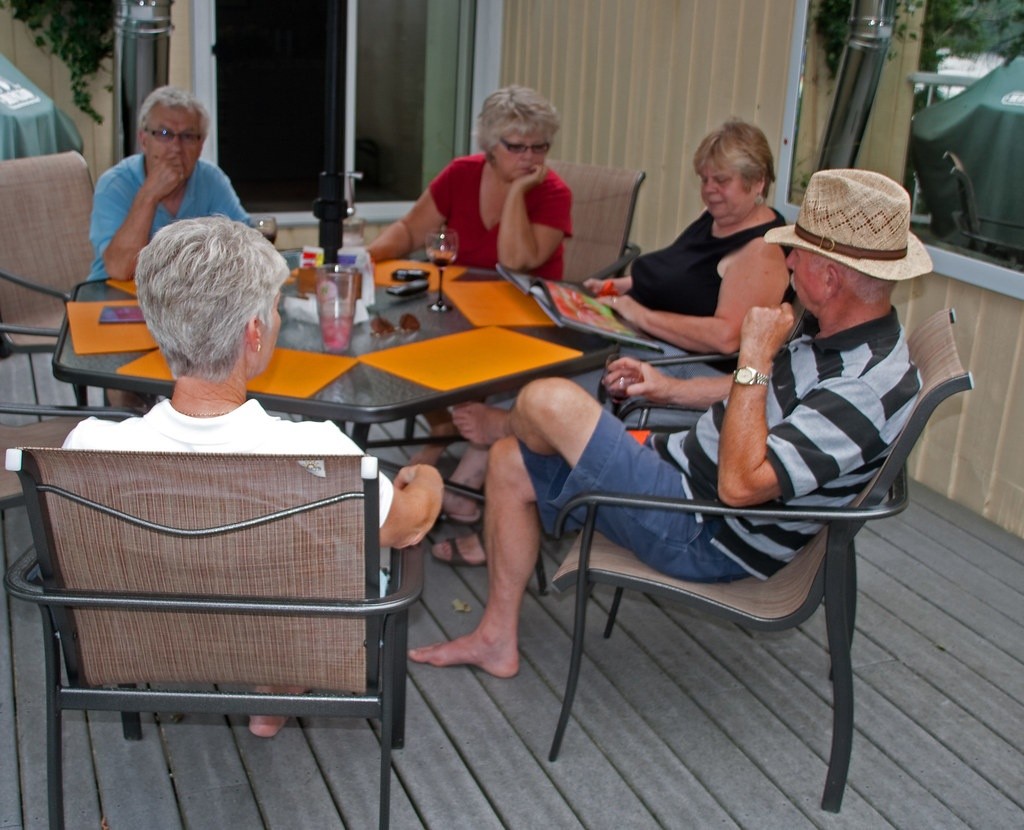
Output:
[496,264,666,352]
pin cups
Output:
[314,273,359,354]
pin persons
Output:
[59,218,444,736]
[364,83,576,466]
[87,83,250,411]
[408,121,934,679]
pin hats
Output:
[764,168,934,281]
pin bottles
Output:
[337,216,370,274]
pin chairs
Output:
[0,149,972,830]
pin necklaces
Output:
[176,409,231,419]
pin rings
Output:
[613,297,618,304]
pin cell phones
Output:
[386,279,429,297]
[391,269,432,282]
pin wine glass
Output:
[249,216,277,245]
[424,228,458,312]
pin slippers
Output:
[433,531,488,565]
[435,504,482,526]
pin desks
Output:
[50,253,623,511]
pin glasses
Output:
[499,137,551,156]
[142,125,203,148]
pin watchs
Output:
[733,364,769,387]
